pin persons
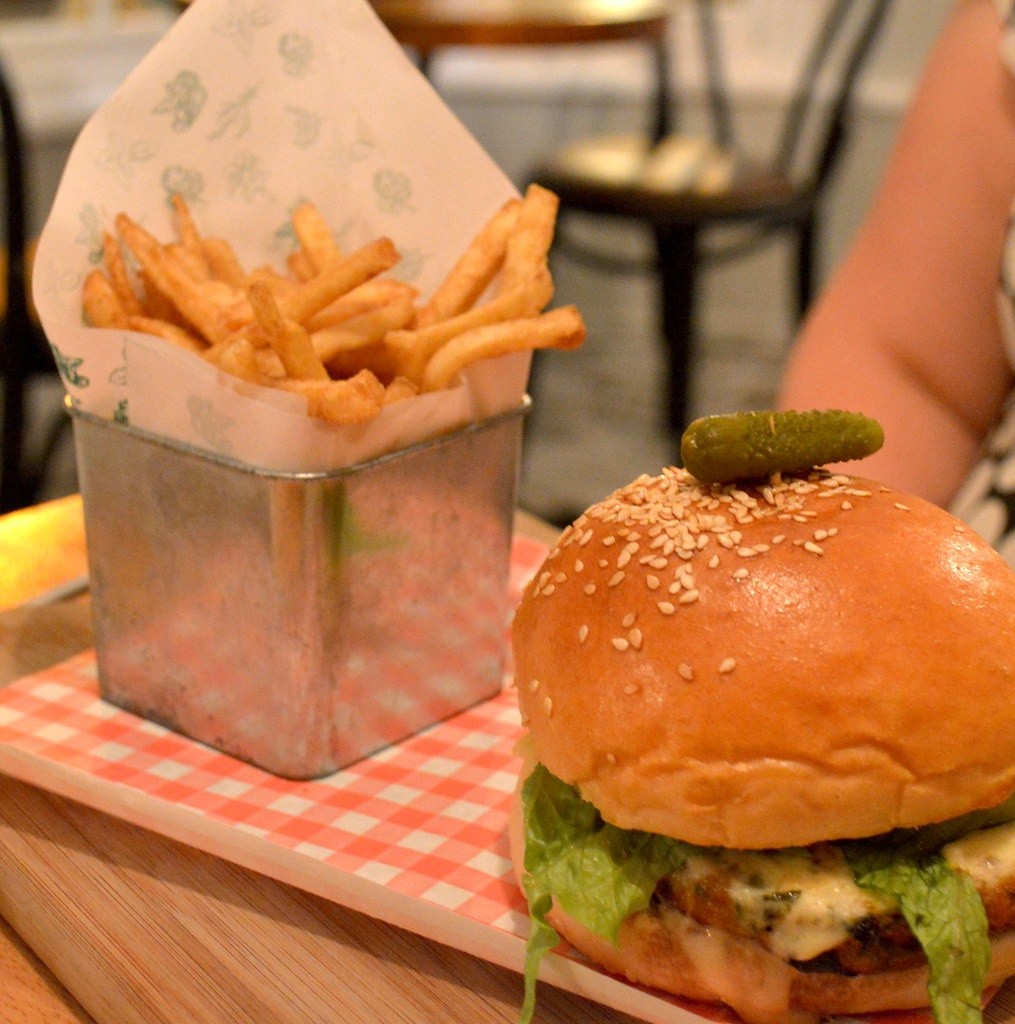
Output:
[775,1,1015,570]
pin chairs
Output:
[525,1,888,455]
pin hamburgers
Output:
[503,461,1015,1023]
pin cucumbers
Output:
[681,409,884,483]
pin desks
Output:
[377,1,673,148]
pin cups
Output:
[62,393,533,780]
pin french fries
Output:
[85,185,584,424]
